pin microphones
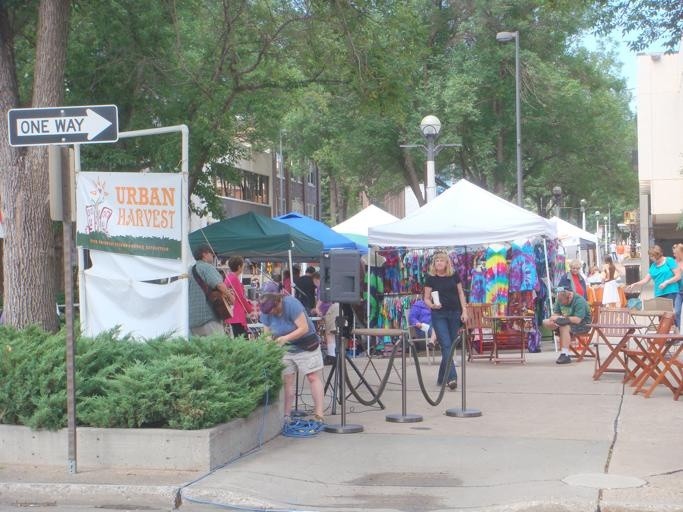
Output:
[290,283,307,298]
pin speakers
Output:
[320,251,360,303]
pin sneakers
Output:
[556,353,571,363]
[557,318,570,325]
[448,381,457,389]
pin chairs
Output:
[562,302,599,363]
[402,308,456,365]
[581,322,648,381]
[592,304,631,362]
[460,303,498,362]
[624,331,682,402]
[622,312,676,396]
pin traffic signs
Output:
[8,104,119,147]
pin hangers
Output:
[380,292,424,303]
[400,248,455,257]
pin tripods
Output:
[324,304,386,415]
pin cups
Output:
[430,291,440,306]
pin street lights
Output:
[399,115,464,203]
[594,210,600,234]
[494,28,524,208]
[602,216,608,253]
[578,198,589,230]
[550,186,564,218]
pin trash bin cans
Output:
[624,265,641,300]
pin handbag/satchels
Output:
[293,334,319,351]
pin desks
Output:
[351,327,408,389]
[625,308,676,359]
[481,315,534,364]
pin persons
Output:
[360,247,385,330]
[670,243,682,304]
[423,253,467,390]
[626,245,681,346]
[408,295,442,352]
[557,259,587,300]
[609,240,618,261]
[601,255,619,319]
[470,236,566,318]
[192,245,356,437]
[541,286,591,364]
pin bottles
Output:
[554,287,566,293]
